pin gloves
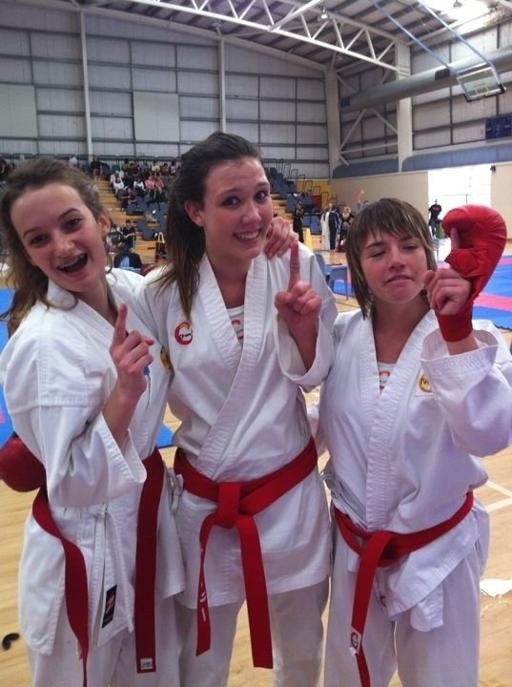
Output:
[0,437,41,492]
[433,204,507,341]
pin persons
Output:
[427,199,442,239]
[0,153,187,687]
[292,199,304,243]
[129,130,341,687]
[119,250,134,269]
[320,189,368,252]
[0,158,16,180]
[69,156,181,252]
[155,241,168,259]
[308,196,512,687]
[143,248,156,256]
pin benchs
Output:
[76,153,328,274]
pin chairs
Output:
[311,250,349,301]
